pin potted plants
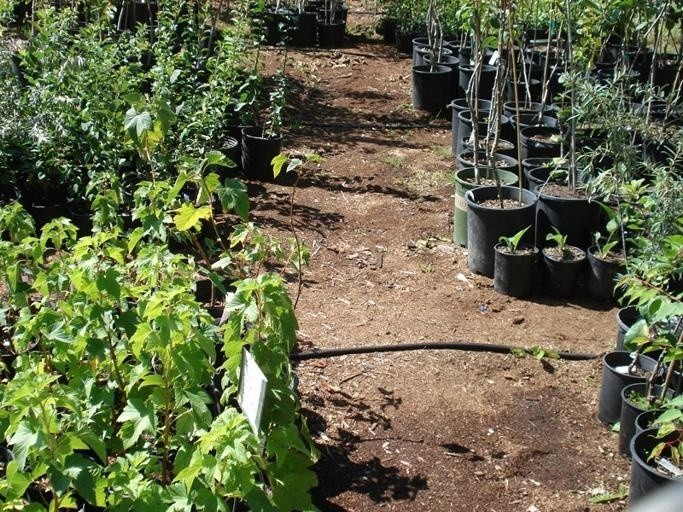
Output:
[1,0,296,512]
[410,0,682,295]
[595,177,681,512]
